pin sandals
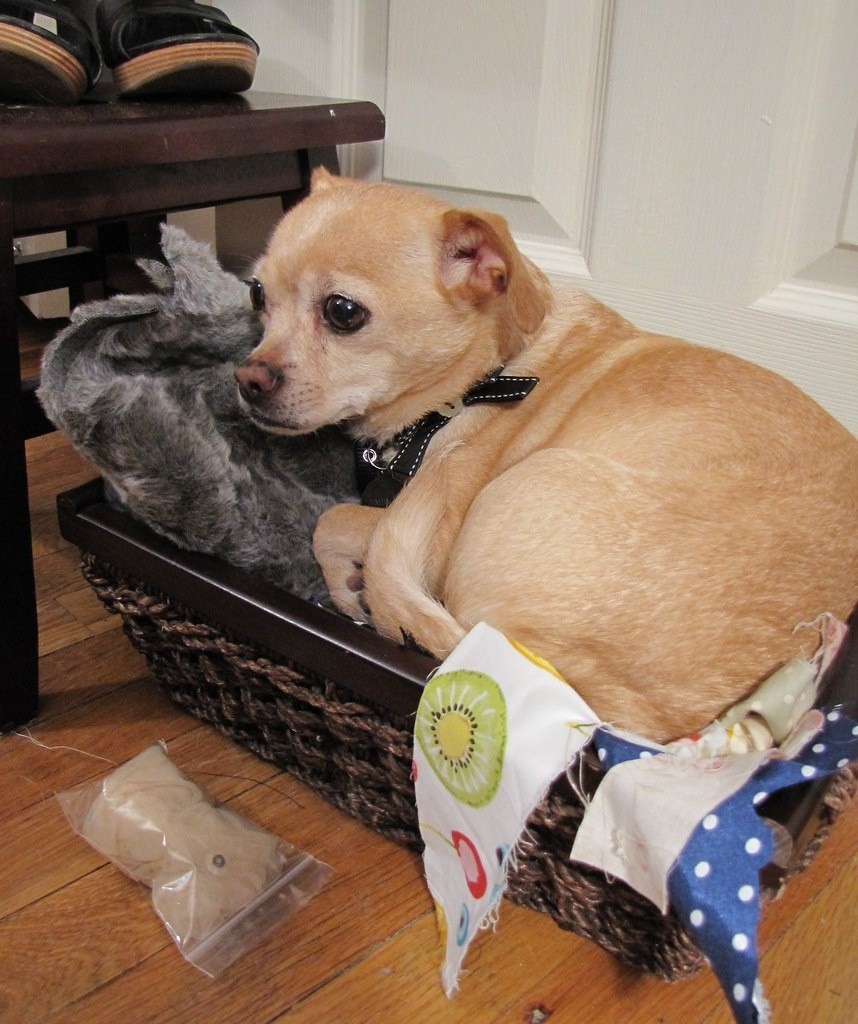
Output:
[96,0,260,97]
[0,0,103,104]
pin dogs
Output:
[231,164,858,746]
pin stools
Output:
[0,82,388,731]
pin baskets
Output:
[56,477,858,985]
